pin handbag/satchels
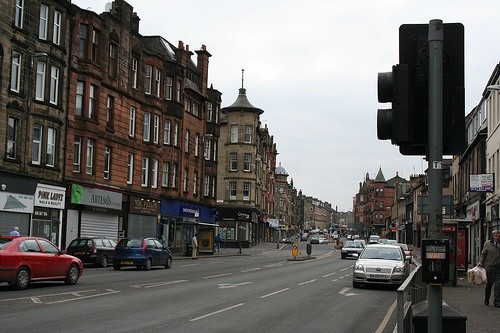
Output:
[469,265,487,285]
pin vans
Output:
[369,235,380,244]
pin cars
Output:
[347,235,360,241]
[64,235,119,269]
[113,236,173,271]
[377,239,389,244]
[352,244,411,292]
[340,241,365,259]
[0,235,84,290]
[301,228,341,245]
[386,240,397,245]
[392,244,414,264]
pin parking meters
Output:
[421,239,449,333]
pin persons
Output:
[215,234,222,252]
[478,229,500,308]
[10,227,19,236]
[192,234,198,260]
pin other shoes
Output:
[494,303,500,308]
[485,300,489,305]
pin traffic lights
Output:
[376,63,412,145]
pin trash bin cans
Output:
[412,315,467,333]
[185,243,192,256]
